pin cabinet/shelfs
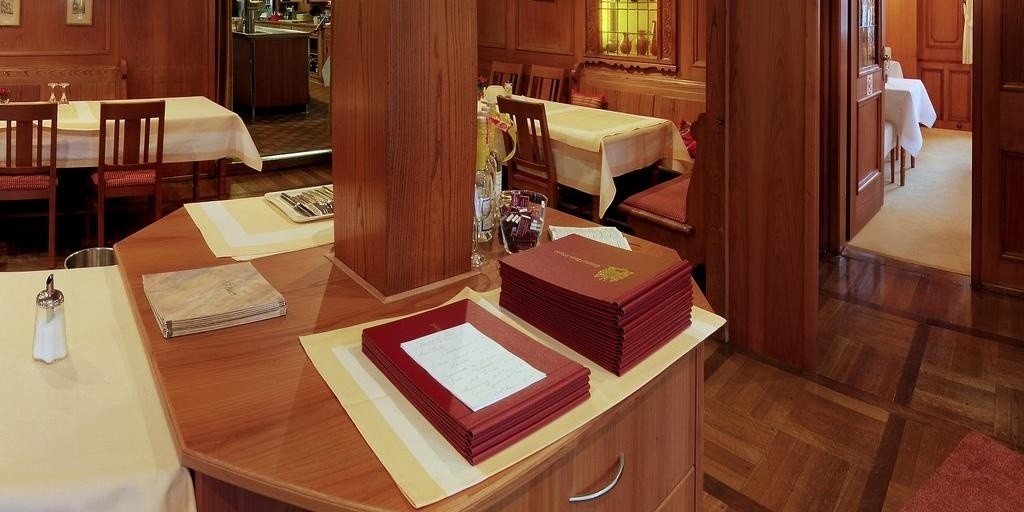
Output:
[477,347,719,512]
[232,19,332,123]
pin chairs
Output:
[88,101,165,246]
[885,121,896,183]
[527,66,567,104]
[885,59,903,159]
[0,104,59,269]
[487,60,526,95]
[497,96,578,208]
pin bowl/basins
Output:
[63,245,118,269]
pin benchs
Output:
[618,113,705,266]
[0,61,127,101]
[570,63,706,133]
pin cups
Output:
[235,20,243,32]
[495,188,549,255]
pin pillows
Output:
[676,118,698,157]
[571,89,605,109]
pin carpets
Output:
[899,429,1024,512]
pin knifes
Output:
[279,183,333,223]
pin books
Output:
[144,287,287,339]
[360,299,591,466]
[141,261,285,328]
[548,225,632,252]
[499,234,694,376]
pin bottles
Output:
[31,272,69,365]
[603,20,657,56]
[470,105,503,267]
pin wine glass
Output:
[46,81,71,104]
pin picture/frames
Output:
[64,0,94,26]
[0,0,20,27]
